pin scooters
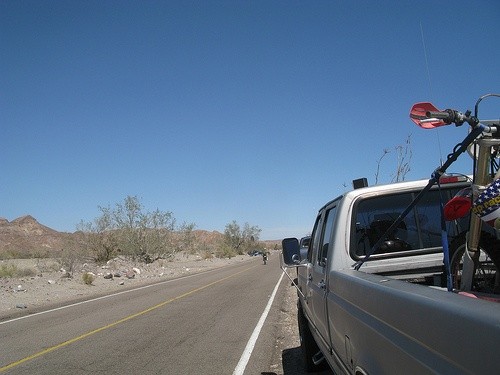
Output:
[263,253,268,265]
[411,96,499,294]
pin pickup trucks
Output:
[280,177,500,374]
